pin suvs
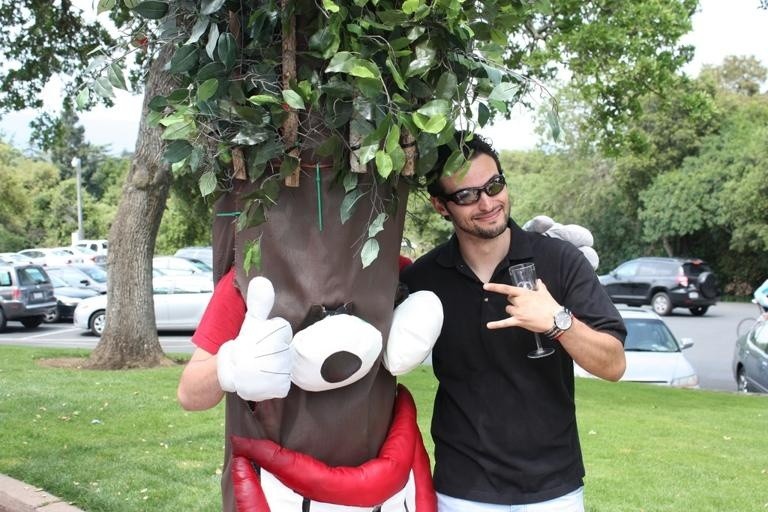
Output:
[596,255,716,316]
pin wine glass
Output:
[510,263,556,359]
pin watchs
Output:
[545,306,573,341]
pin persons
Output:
[97,1,599,512]
[398,130,626,512]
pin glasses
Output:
[440,174,506,205]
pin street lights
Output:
[70,157,84,229]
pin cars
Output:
[572,309,768,391]
[1,240,212,338]
